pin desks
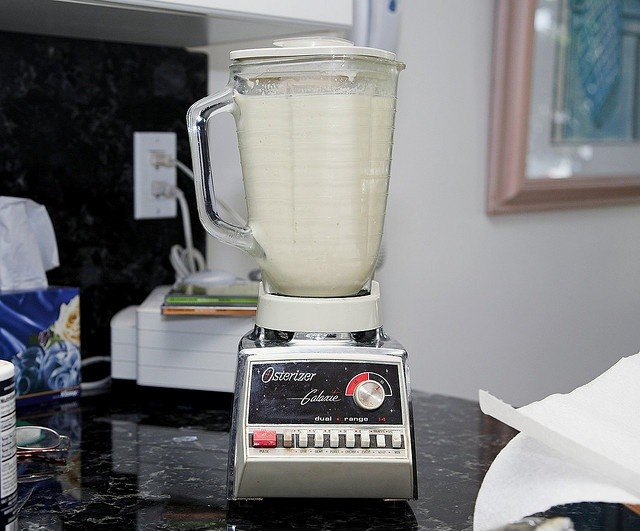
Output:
[0,378,640,531]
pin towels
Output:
[466,515,573,531]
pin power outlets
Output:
[132,131,179,220]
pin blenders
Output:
[186,37,417,503]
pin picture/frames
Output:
[484,0,640,215]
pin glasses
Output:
[15,426,71,465]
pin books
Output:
[160,288,258,318]
